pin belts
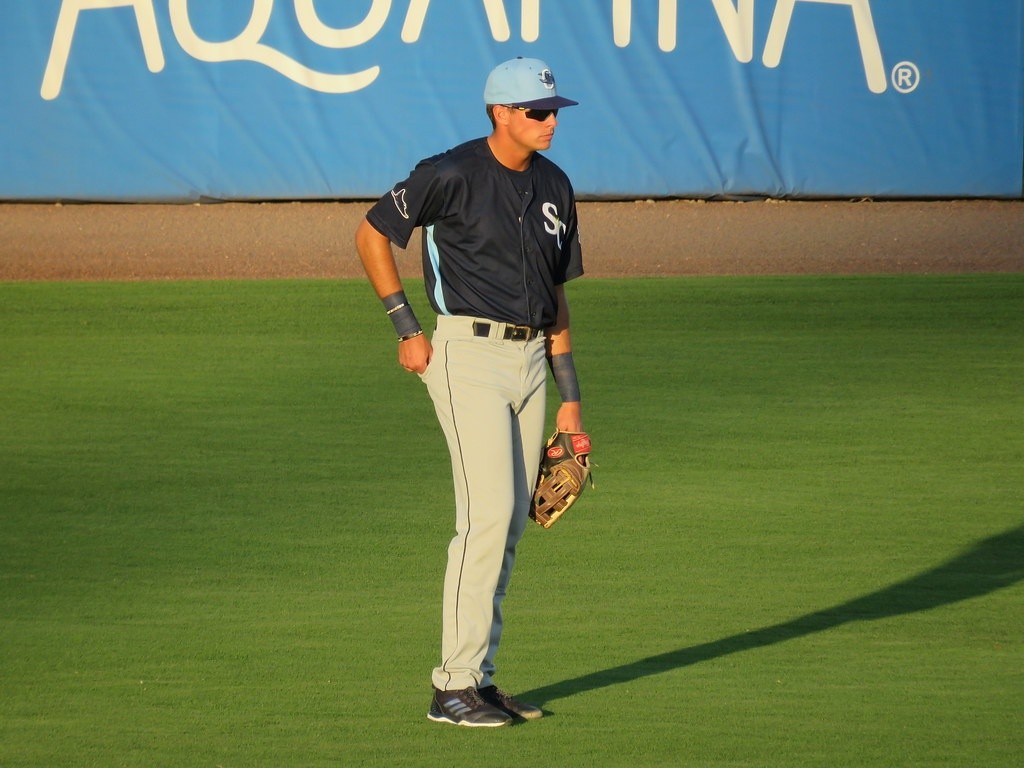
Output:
[433,318,540,342]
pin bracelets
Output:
[397,330,422,343]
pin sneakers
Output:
[426,684,544,727]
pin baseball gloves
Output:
[527,427,597,531]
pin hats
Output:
[484,56,579,110]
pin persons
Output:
[356,58,591,727]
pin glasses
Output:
[509,106,559,122]
